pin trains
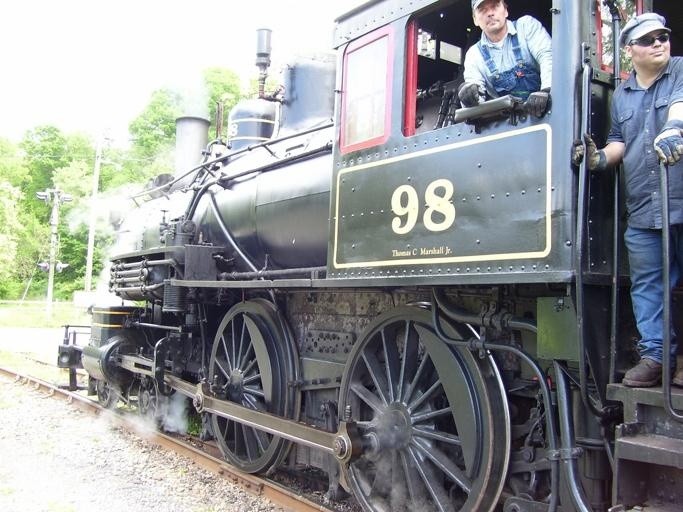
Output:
[55,0,682,512]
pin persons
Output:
[457,0,552,118]
[572,13,683,388]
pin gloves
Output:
[654,119,683,166]
[457,82,480,107]
[572,134,607,171]
[522,91,548,118]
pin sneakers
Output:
[622,358,683,389]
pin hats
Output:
[619,12,672,48]
[470,0,485,10]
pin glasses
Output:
[632,33,670,44]
